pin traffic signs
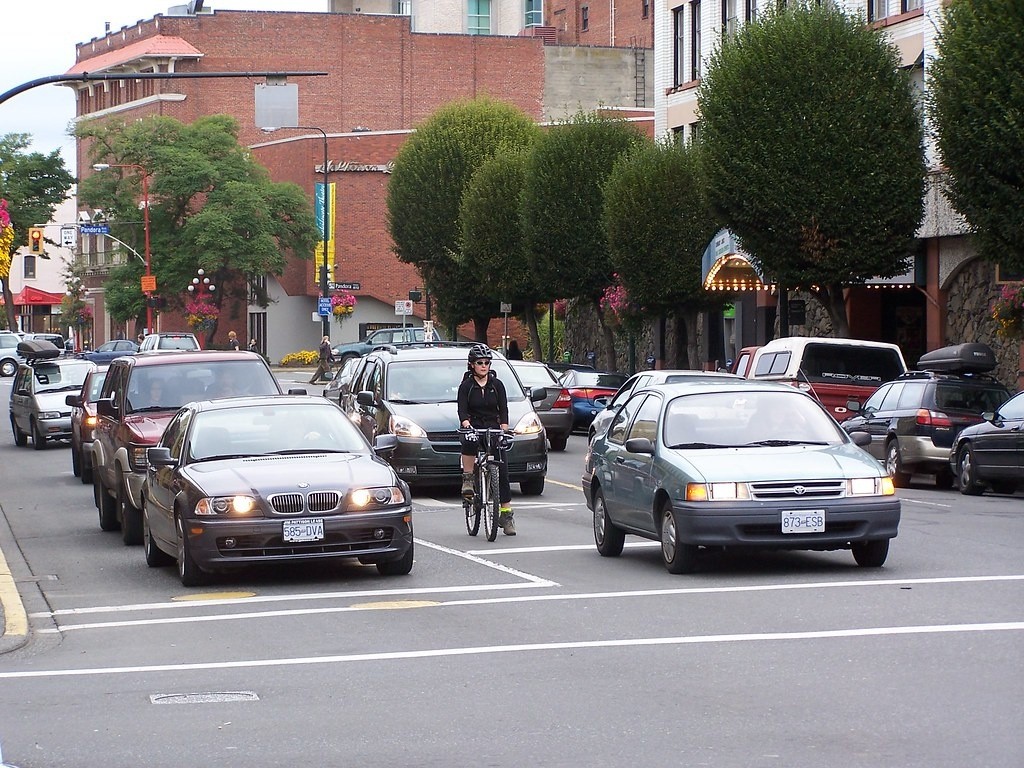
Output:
[60,226,75,246]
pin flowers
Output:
[77,306,92,329]
[555,298,567,320]
[145,290,165,317]
[599,272,648,339]
[182,292,220,331]
[331,294,357,328]
[991,279,1024,337]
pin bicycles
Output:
[455,423,516,542]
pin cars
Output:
[65,365,139,484]
[581,378,901,575]
[10,340,98,451]
[73,340,141,366]
[503,359,632,453]
[948,390,1024,496]
[322,357,363,410]
[141,393,414,588]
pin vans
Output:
[727,337,909,426]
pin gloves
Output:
[464,424,479,441]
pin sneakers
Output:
[462,473,475,494]
[498,509,517,536]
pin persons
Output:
[249,339,258,352]
[138,334,144,344]
[457,344,517,536]
[228,331,239,351]
[508,341,523,360]
[148,378,163,401]
[310,335,335,385]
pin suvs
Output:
[332,327,444,369]
[0,330,74,378]
[343,342,549,496]
[135,332,202,355]
[92,350,308,548]
[840,342,1013,490]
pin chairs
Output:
[631,413,699,446]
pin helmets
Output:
[468,343,492,362]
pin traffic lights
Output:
[28,228,44,254]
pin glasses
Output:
[470,361,490,365]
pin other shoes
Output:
[309,381,317,385]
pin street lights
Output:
[92,163,152,335]
[260,126,329,337]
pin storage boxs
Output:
[917,343,997,376]
[17,339,59,358]
